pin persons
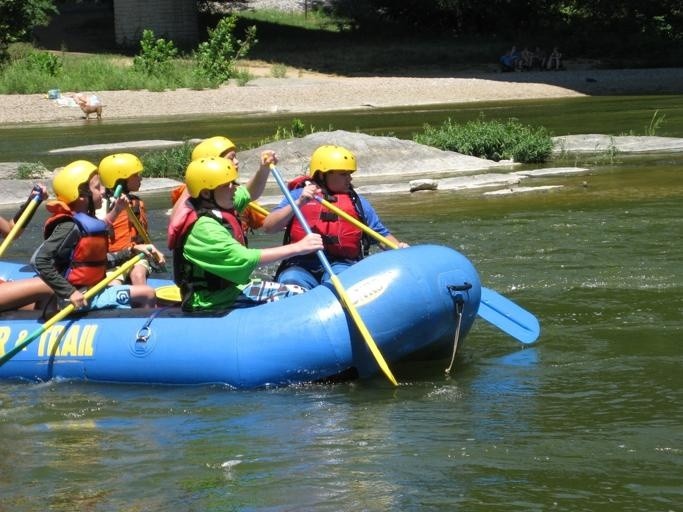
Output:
[174,151,324,313]
[98,154,165,286]
[31,162,156,308]
[262,145,407,291]
[191,134,266,253]
[0,183,56,310]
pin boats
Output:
[0,244,481,389]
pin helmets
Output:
[52,159,98,205]
[98,153,144,189]
[309,144,357,179]
[185,156,238,199]
[191,136,236,162]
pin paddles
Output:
[270,162,398,390]
[311,190,541,344]
